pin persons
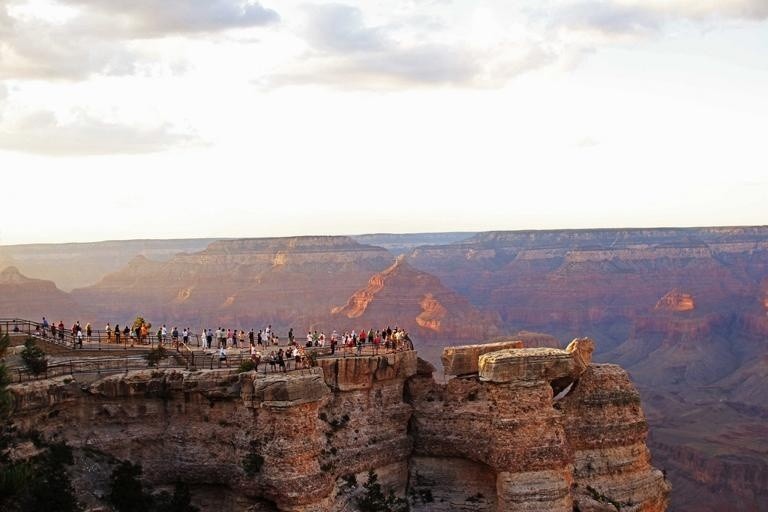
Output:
[330,326,408,355]
[105,322,150,346]
[247,325,286,372]
[201,327,245,367]
[286,328,326,370]
[157,324,193,345]
[36,317,91,349]
